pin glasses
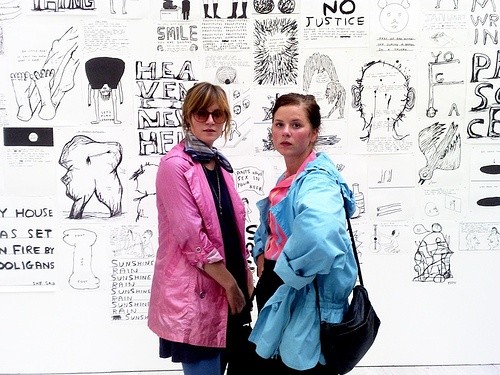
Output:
[192,108,228,123]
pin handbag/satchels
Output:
[319,286,381,375]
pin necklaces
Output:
[202,162,223,215]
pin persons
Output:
[148,81,254,375]
[251,93,359,375]
